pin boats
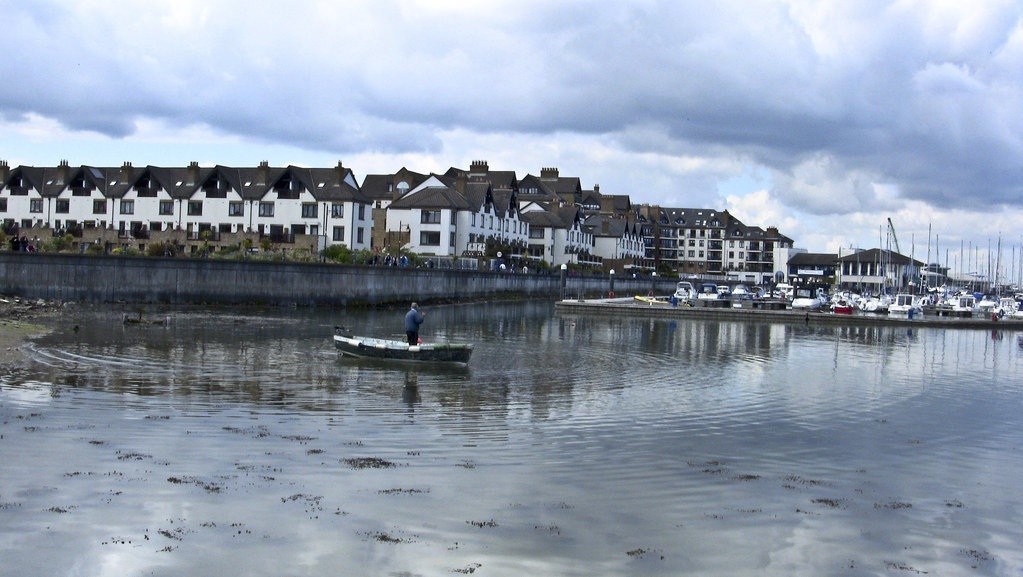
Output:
[331,330,475,368]
[634,279,861,315]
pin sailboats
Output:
[864,215,1023,322]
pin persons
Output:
[404,303,424,346]
[385,252,409,267]
